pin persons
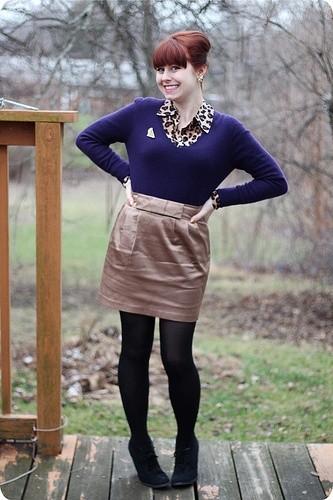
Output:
[73,29,291,491]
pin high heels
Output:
[169,435,200,487]
[129,434,169,488]
